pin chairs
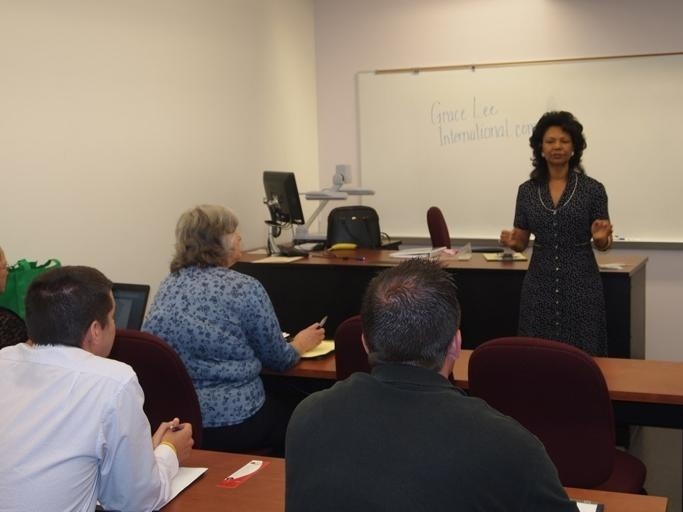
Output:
[332,315,457,386]
[427,205,504,253]
[109,283,150,330]
[326,206,380,250]
[105,328,203,448]
[466,335,650,496]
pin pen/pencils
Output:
[171,424,185,433]
[316,316,328,329]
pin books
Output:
[391,245,447,259]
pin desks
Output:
[293,236,402,250]
[156,437,671,511]
[278,341,683,428]
[234,247,646,359]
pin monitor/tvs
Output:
[262,170,306,227]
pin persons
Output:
[283,258,578,509]
[0,246,28,352]
[144,203,329,457]
[1,265,195,511]
[499,110,615,360]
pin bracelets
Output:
[598,237,611,252]
[159,441,178,456]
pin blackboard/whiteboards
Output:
[354,51,683,252]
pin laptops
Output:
[97,283,150,334]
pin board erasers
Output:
[502,253,513,261]
